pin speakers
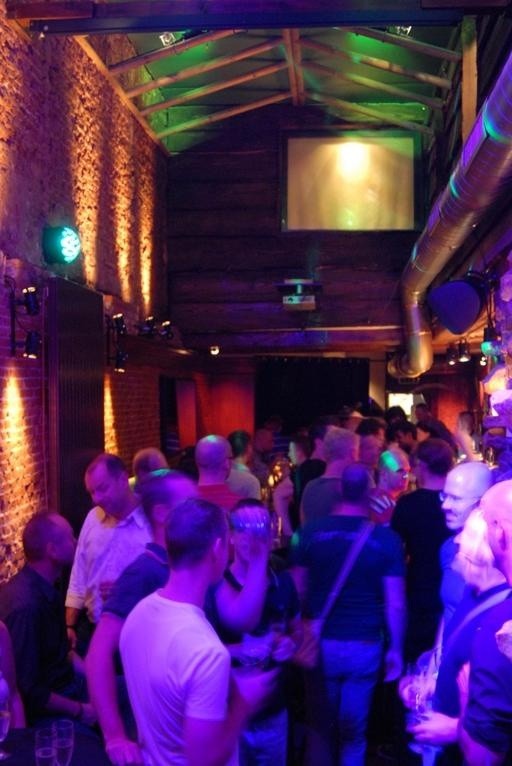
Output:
[427,280,483,334]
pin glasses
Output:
[440,493,480,501]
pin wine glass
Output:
[0,696,76,766]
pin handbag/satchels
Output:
[288,618,324,669]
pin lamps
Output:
[44,223,80,263]
[115,354,127,372]
[162,320,175,340]
[457,341,471,363]
[481,326,497,357]
[427,272,488,335]
[21,286,40,315]
[447,346,457,366]
[113,313,126,335]
[23,330,42,361]
[145,316,155,326]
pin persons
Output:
[0,397,512,766]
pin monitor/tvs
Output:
[280,129,424,235]
